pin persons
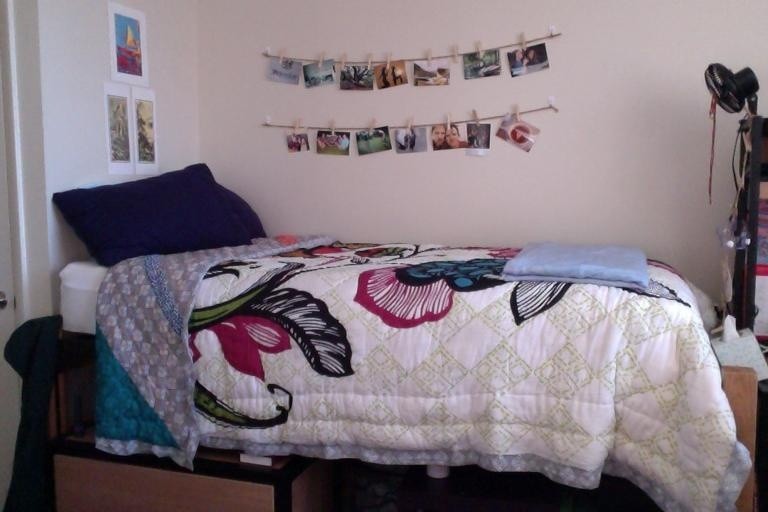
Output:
[379,67,390,88]
[391,65,402,85]
[512,50,528,74]
[432,125,450,150]
[446,126,468,148]
[318,134,349,150]
[527,48,539,64]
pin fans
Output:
[704,59,761,116]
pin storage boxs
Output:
[41,428,344,510]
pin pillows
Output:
[51,162,268,269]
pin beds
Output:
[58,233,706,482]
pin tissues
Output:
[710,313,767,382]
